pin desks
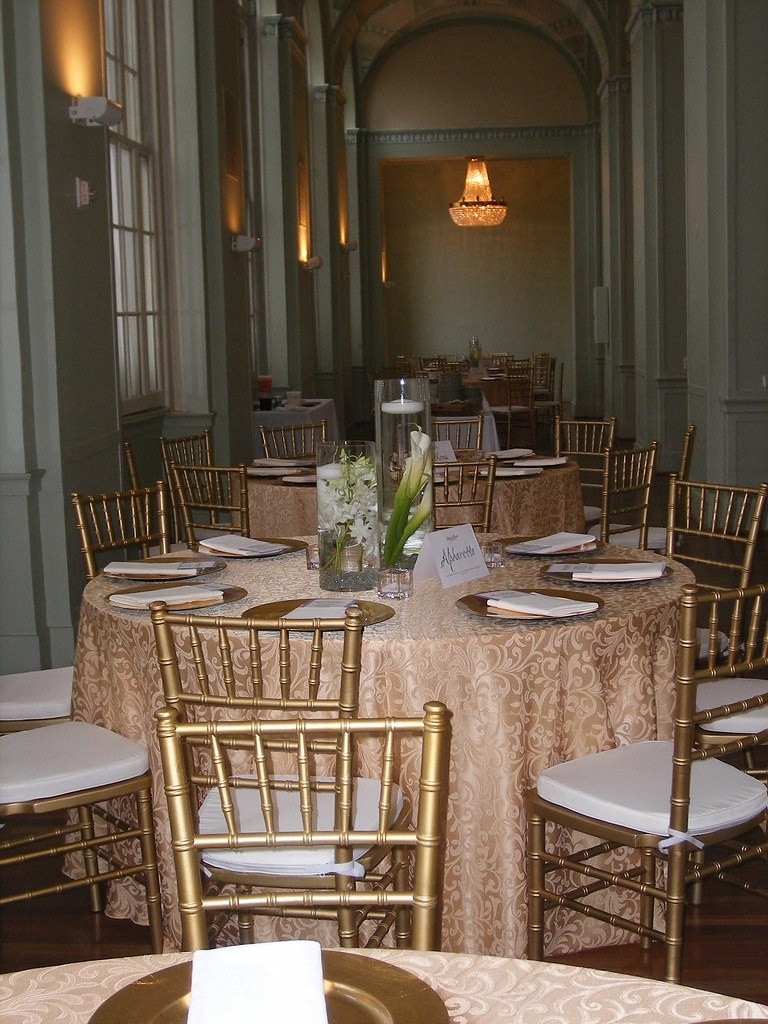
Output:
[253,398,338,456]
[63,531,694,960]
[0,942,768,1024]
[432,375,530,407]
[439,366,523,376]
[228,453,588,538]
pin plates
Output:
[417,361,508,381]
[455,589,605,619]
[539,559,673,584]
[490,535,604,556]
[104,581,248,613]
[105,556,227,580]
[88,947,452,1024]
[240,598,395,632]
[239,441,567,486]
[190,537,309,557]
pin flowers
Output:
[382,424,433,568]
[470,344,480,364]
[318,447,379,575]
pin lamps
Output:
[303,257,324,271]
[449,155,508,226]
[231,234,261,252]
[68,95,124,126]
[340,240,357,250]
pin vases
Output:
[375,379,434,599]
[469,337,479,366]
[315,440,378,591]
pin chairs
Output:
[0,352,768,954]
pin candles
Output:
[257,376,272,400]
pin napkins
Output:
[281,604,359,620]
[252,458,312,467]
[187,939,328,1024]
[486,592,598,617]
[283,475,316,482]
[512,456,568,466]
[506,533,597,553]
[482,448,533,460]
[110,585,224,606]
[199,535,289,556]
[573,560,665,580]
[245,467,301,475]
[480,467,543,476]
[104,560,202,576]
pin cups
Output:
[286,391,302,407]
[478,539,507,568]
[377,567,413,599]
[258,375,273,410]
